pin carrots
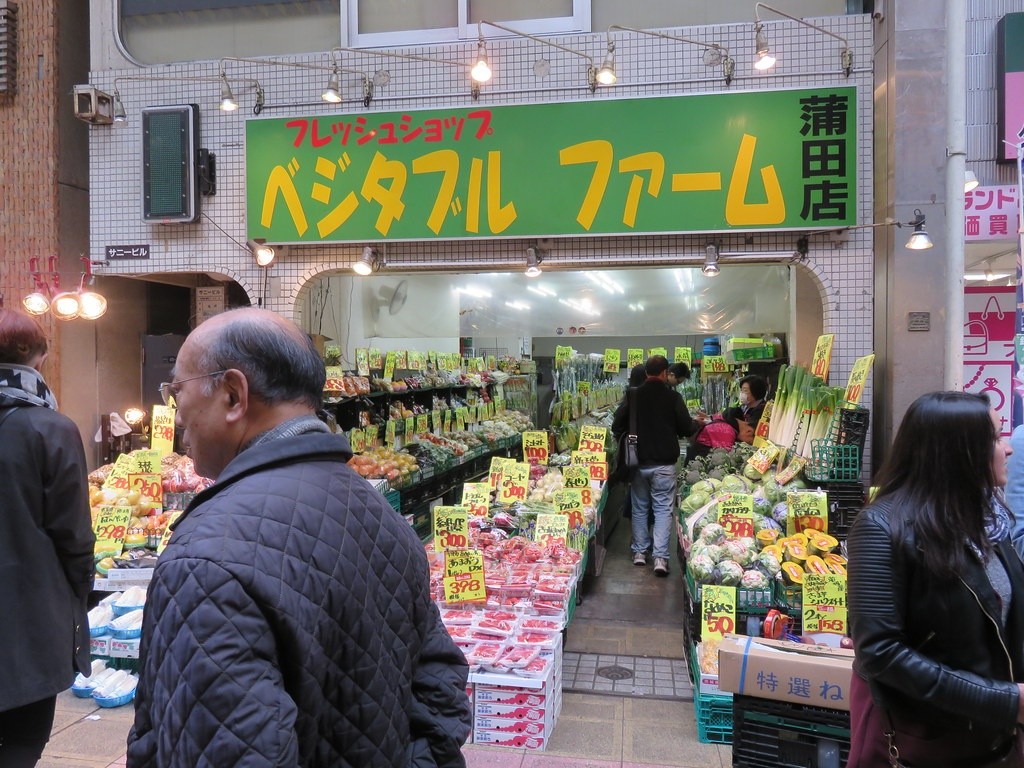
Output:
[344,376,370,394]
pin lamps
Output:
[701,239,723,277]
[50,270,108,322]
[125,406,150,443]
[321,46,479,102]
[113,76,264,121]
[752,3,855,79]
[352,247,380,277]
[964,169,980,194]
[469,19,598,95]
[984,258,997,282]
[524,247,544,278]
[219,56,371,112]
[594,25,737,87]
[842,208,934,251]
[23,270,60,316]
[246,240,275,268]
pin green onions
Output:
[768,364,845,478]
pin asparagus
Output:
[567,526,589,550]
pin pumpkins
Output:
[757,527,849,587]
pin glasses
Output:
[676,377,683,384]
[157,372,229,406]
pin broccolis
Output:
[531,472,563,503]
[686,523,781,589]
[684,442,756,484]
[487,500,521,518]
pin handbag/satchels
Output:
[869,720,1024,768]
[616,431,638,483]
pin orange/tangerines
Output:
[345,455,400,482]
[88,486,151,568]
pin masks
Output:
[740,393,753,403]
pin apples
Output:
[362,448,418,470]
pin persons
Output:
[739,375,767,425]
[684,406,756,468]
[846,391,1024,768]
[612,356,706,575]
[126,309,471,768]
[0,307,96,768]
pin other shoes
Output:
[630,552,647,564]
[653,557,671,575]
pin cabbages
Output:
[678,465,807,538]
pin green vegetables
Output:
[520,513,538,541]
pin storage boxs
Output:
[691,352,703,359]
[519,361,537,373]
[692,360,702,363]
[92,374,870,768]
[726,337,765,352]
[724,346,775,366]
